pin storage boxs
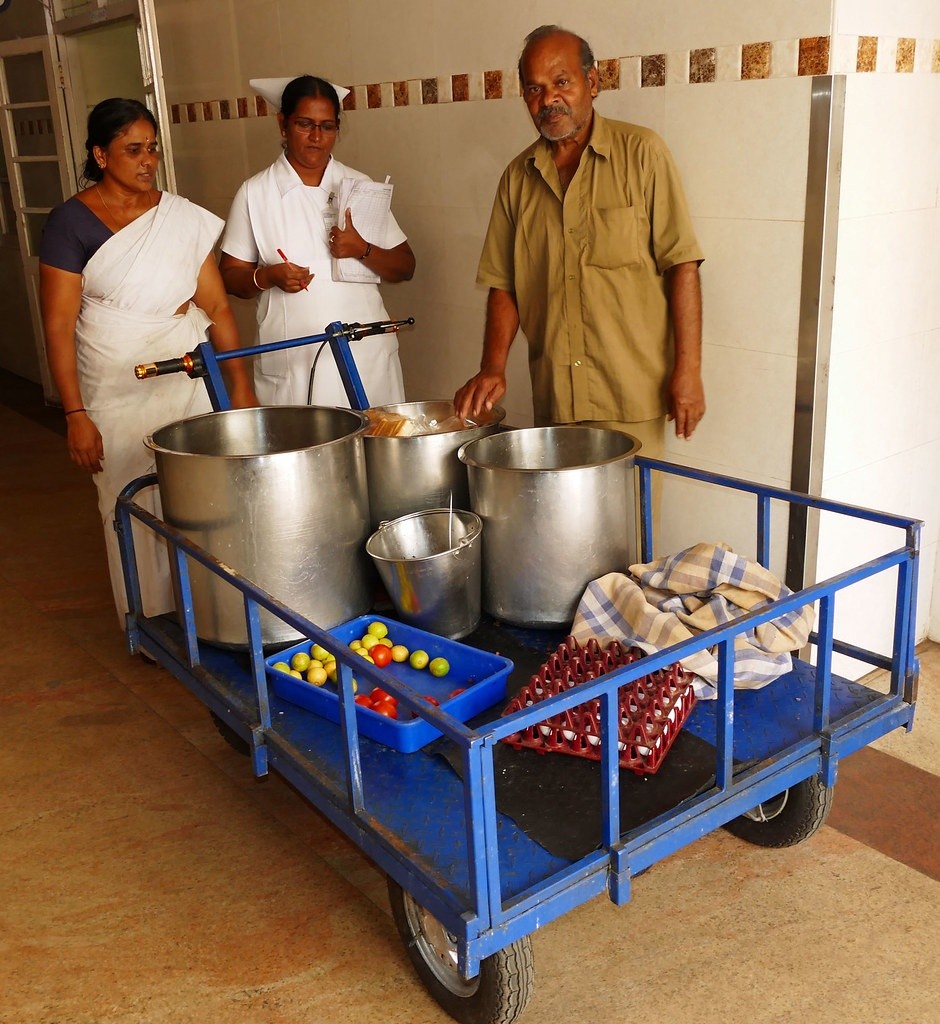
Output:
[263,615,513,754]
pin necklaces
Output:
[95,184,152,229]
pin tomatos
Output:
[352,644,467,719]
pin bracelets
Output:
[357,243,371,260]
[65,409,86,416]
[253,268,270,291]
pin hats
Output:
[249,76,350,113]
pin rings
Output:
[329,236,335,243]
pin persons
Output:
[40,97,260,668]
[219,74,414,409]
[454,24,705,564]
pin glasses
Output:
[286,116,340,135]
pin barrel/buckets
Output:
[458,425,642,624]
[143,405,370,650]
[363,400,506,530]
[365,490,483,640]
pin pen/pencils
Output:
[276,247,310,293]
